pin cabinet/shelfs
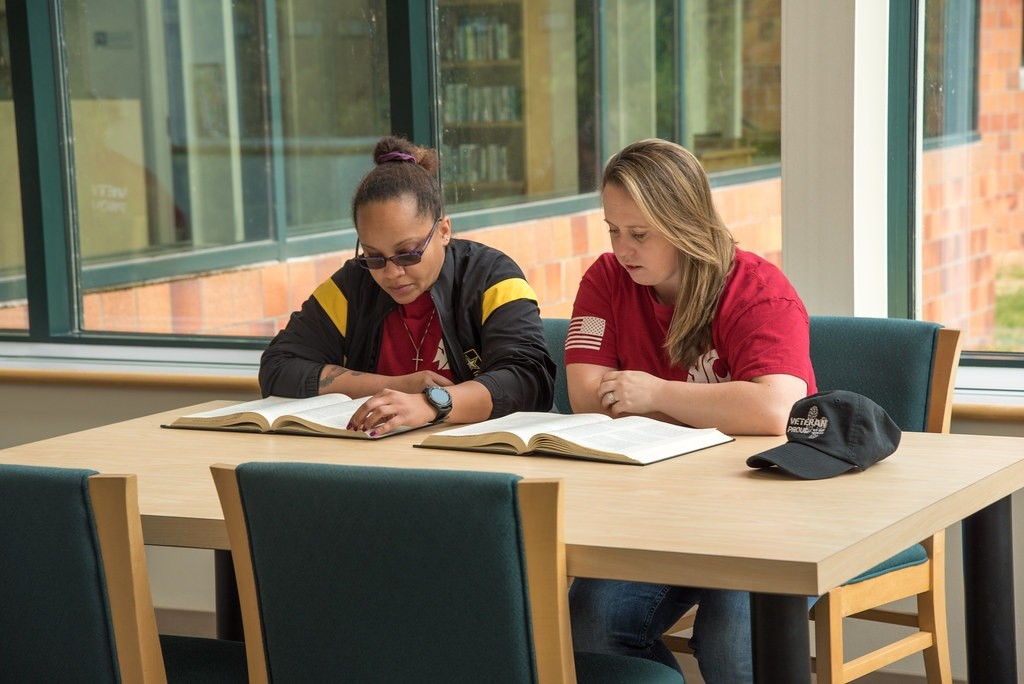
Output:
[656,0,711,159]
[60,0,247,257]
[1,97,152,269]
[429,0,577,206]
[576,0,657,193]
[233,0,392,238]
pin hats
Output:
[746,390,901,480]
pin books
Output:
[441,11,511,63]
[412,411,737,466]
[160,392,435,441]
[442,142,508,181]
[441,82,517,124]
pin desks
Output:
[0,400,1024,684]
[691,146,759,173]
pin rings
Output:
[608,392,616,404]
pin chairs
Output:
[210,460,576,684]
[536,311,572,600]
[0,462,167,684]
[642,314,961,684]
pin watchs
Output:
[422,386,453,425]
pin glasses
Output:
[355,217,443,269]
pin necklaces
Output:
[404,308,436,371]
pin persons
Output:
[566,136,821,684]
[258,135,557,437]
